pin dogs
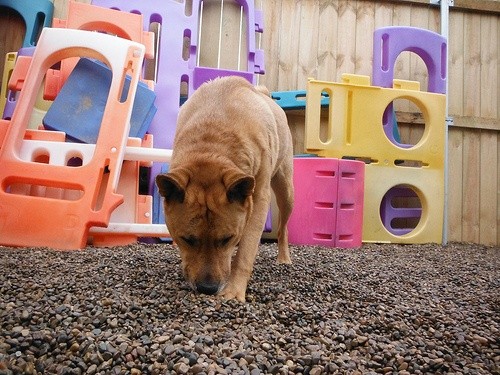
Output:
[155,75,294,302]
